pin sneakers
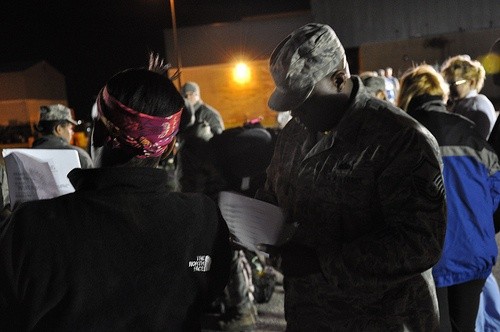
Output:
[211,312,257,331]
[226,304,258,321]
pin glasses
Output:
[447,78,467,86]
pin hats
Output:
[39,103,78,126]
[183,82,199,93]
[267,23,345,112]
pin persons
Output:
[0,67,232,332]
[175,82,225,145]
[254,22,448,332]
[2,104,96,206]
[357,39,500,332]
